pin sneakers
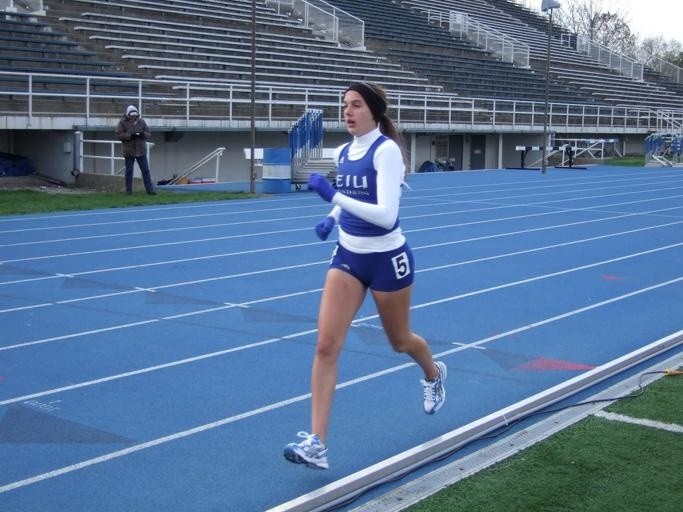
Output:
[420,361,447,415]
[283,431,329,470]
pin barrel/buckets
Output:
[262,148,292,194]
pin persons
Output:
[283,82,449,474]
[117,104,157,196]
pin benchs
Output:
[0,0,683,127]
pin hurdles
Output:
[289,107,337,185]
[645,134,682,169]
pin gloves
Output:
[307,172,337,203]
[131,131,144,140]
[315,216,336,242]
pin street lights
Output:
[540,0,561,174]
